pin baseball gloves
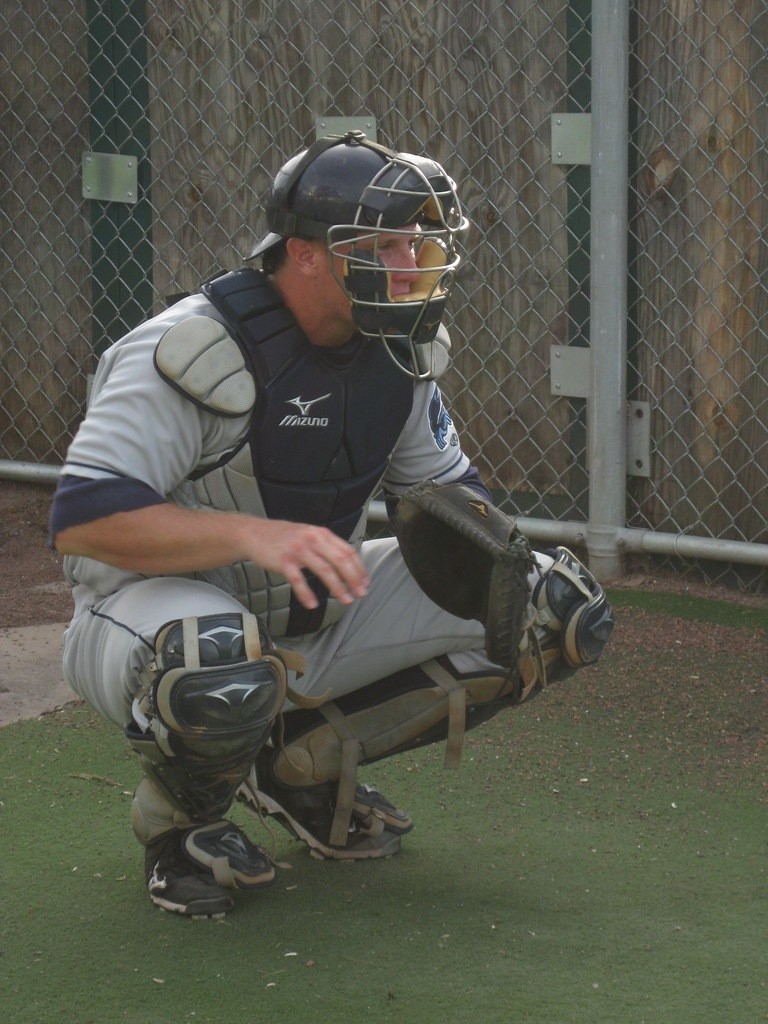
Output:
[394,473,534,676]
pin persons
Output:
[49,129,615,918]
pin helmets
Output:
[242,136,457,262]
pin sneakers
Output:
[144,820,234,920]
[235,754,413,858]
